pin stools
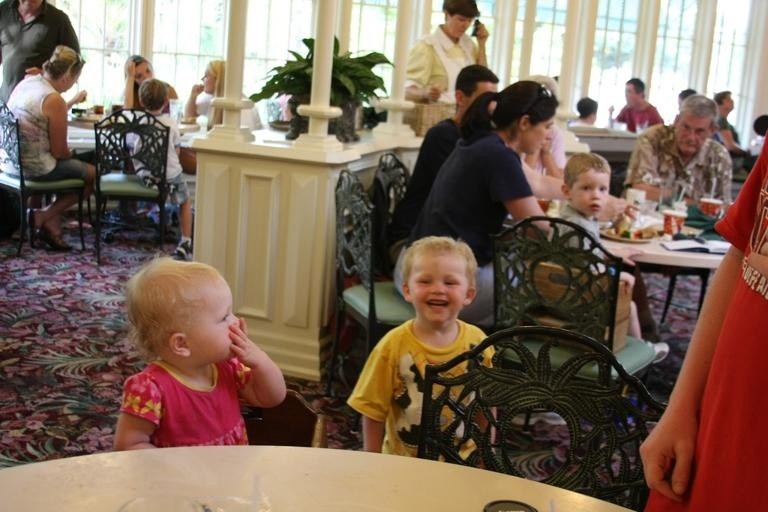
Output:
[649,266,711,330]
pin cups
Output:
[662,209,686,241]
[636,198,657,220]
[635,118,649,135]
[700,197,723,219]
[169,98,182,125]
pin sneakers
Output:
[173,240,192,260]
[645,341,669,365]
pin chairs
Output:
[413,324,666,512]
[487,216,661,419]
[372,153,412,224]
[1,101,87,257]
[94,108,169,264]
[323,169,418,402]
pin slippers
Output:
[36,228,74,252]
[26,206,41,249]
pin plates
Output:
[599,226,658,244]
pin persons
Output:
[112,254,286,450]
[346,232,496,472]
[1,1,225,259]
[399,0,768,512]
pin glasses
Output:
[520,82,553,117]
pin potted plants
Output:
[245,31,395,144]
